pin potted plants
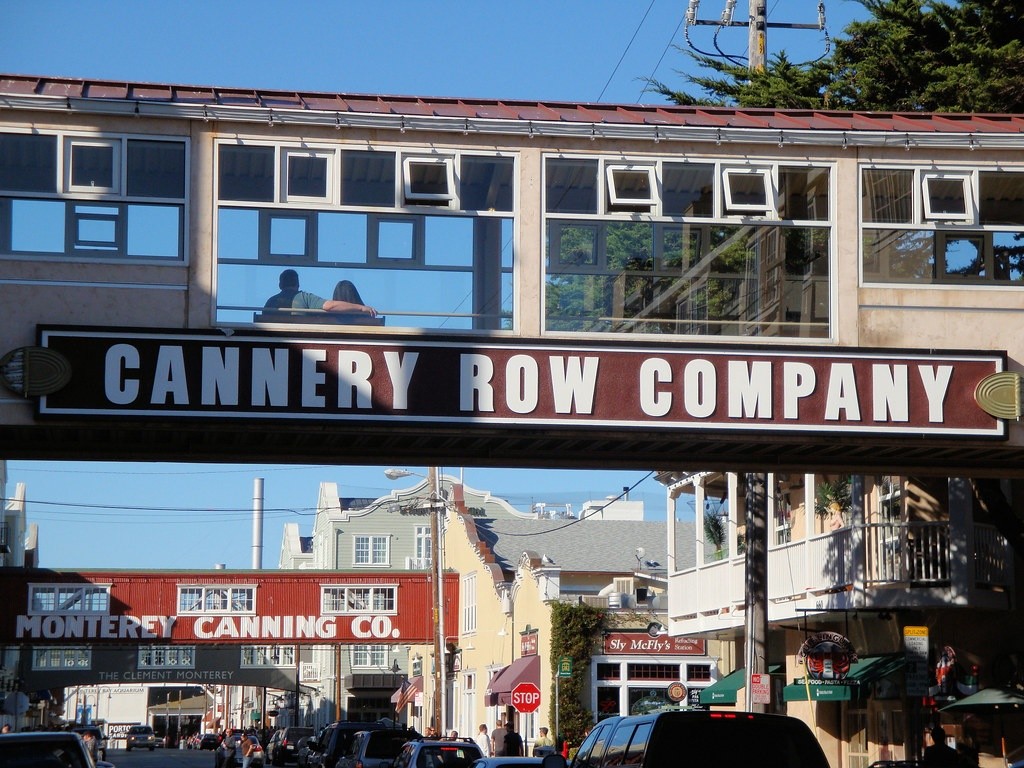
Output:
[813,475,853,593]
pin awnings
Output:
[484,655,541,708]
[698,663,784,705]
[390,676,424,703]
[783,653,907,702]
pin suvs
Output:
[379,737,484,768]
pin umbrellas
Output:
[938,681,1024,768]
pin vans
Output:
[568,711,831,768]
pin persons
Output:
[533,727,554,757]
[561,737,569,760]
[503,721,524,757]
[304,720,353,742]
[402,726,459,741]
[585,728,591,737]
[923,725,960,768]
[490,720,508,757]
[0,722,100,768]
[221,725,291,768]
[476,724,491,758]
[262,269,378,318]
[187,732,203,750]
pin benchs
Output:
[253,312,386,326]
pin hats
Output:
[539,727,548,734]
[504,722,514,727]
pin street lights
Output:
[200,686,208,734]
[208,683,218,735]
[95,686,113,725]
[384,469,447,740]
[83,695,96,726]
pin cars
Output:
[154,738,164,748]
[265,726,317,764]
[467,756,572,768]
[334,730,425,768]
[124,726,155,751]
[192,734,219,751]
[215,729,244,748]
[304,720,387,768]
[215,733,264,768]
[0,732,116,768]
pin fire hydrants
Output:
[561,741,569,759]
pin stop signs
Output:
[510,682,541,713]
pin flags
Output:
[394,678,418,714]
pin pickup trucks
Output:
[72,726,108,760]
